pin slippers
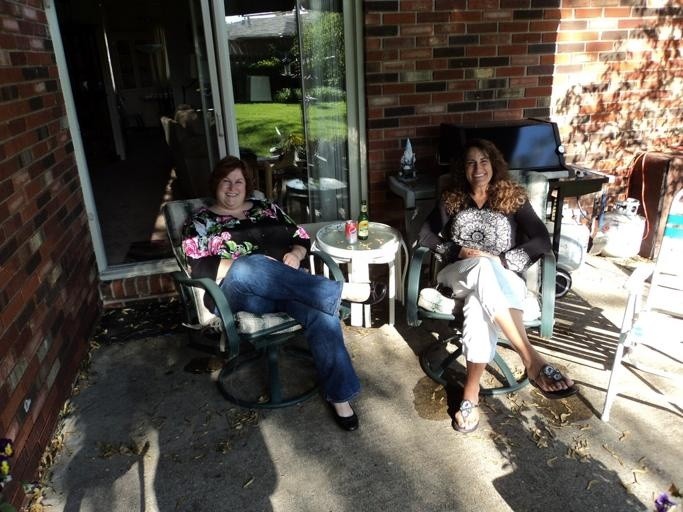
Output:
[528,364,579,399]
[451,398,480,434]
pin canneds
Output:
[345,220,359,244]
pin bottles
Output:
[358,200,369,240]
[313,160,321,187]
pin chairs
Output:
[161,188,350,411]
[406,169,558,394]
[601,188,683,423]
[117,93,147,141]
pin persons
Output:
[181,154,388,433]
[415,137,578,434]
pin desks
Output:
[387,163,609,264]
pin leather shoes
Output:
[341,281,387,305]
[327,400,359,432]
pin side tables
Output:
[309,220,410,328]
[282,177,347,224]
